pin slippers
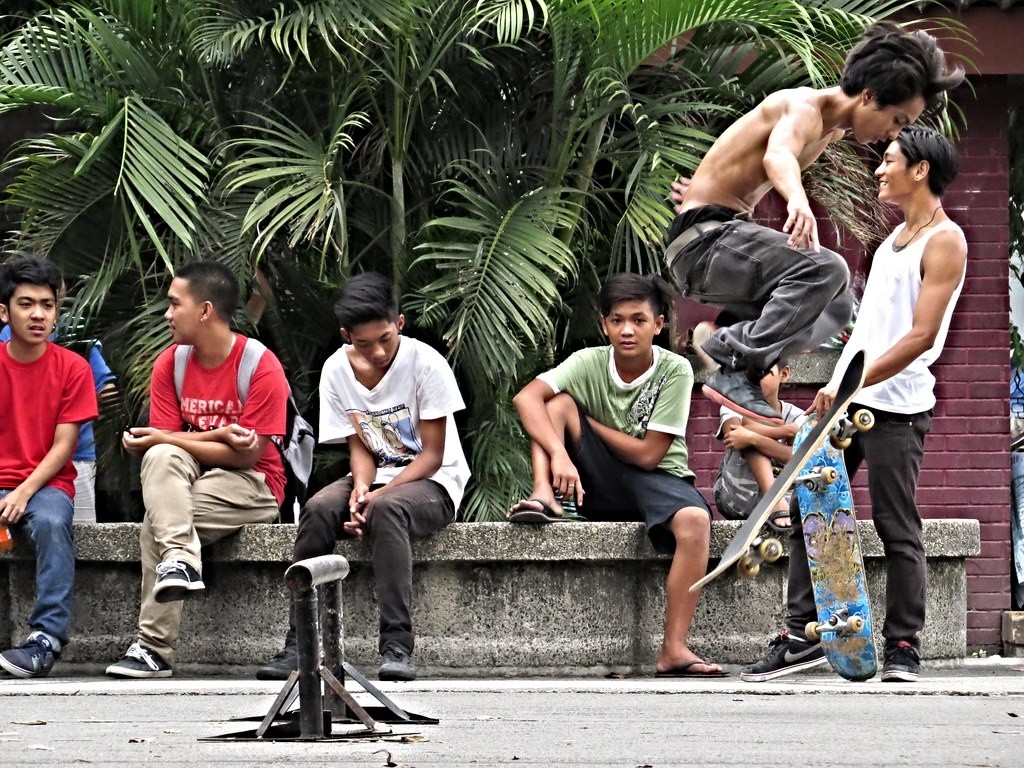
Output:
[655,661,730,677]
[765,510,793,532]
[509,499,571,523]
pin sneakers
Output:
[154,560,206,603]
[378,647,417,680]
[691,321,720,370]
[881,641,921,681]
[702,367,784,428]
[106,643,172,677]
[255,651,298,679]
[1,632,62,679]
[740,635,828,682]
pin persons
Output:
[735,122,973,684]
[656,17,968,433]
[102,258,292,679]
[251,272,478,684]
[500,271,736,679]
[710,351,821,535]
[0,257,97,679]
[0,258,115,525]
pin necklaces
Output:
[609,352,655,434]
[891,203,943,252]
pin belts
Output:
[665,220,723,268]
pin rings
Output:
[568,483,574,488]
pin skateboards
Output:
[791,408,879,681]
[687,349,875,596]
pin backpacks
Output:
[174,336,316,503]
